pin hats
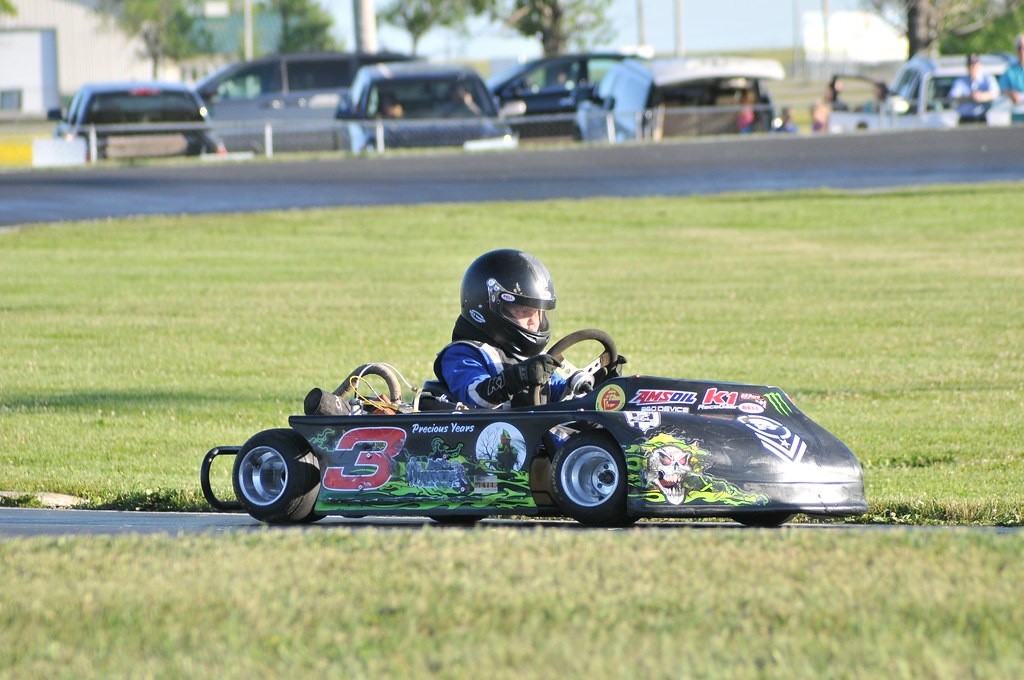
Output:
[966,51,978,66]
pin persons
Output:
[381,100,403,118]
[948,52,1002,124]
[443,85,481,115]
[865,83,888,113]
[812,99,826,129]
[738,91,756,133]
[999,33,1024,122]
[434,249,627,411]
[551,72,567,88]
[770,109,798,133]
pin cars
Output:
[47,82,229,163]
[331,62,520,159]
[569,55,798,140]
[484,50,642,117]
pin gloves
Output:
[518,354,562,389]
[590,354,628,389]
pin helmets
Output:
[459,248,557,361]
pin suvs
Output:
[193,49,447,153]
[825,49,1020,133]
